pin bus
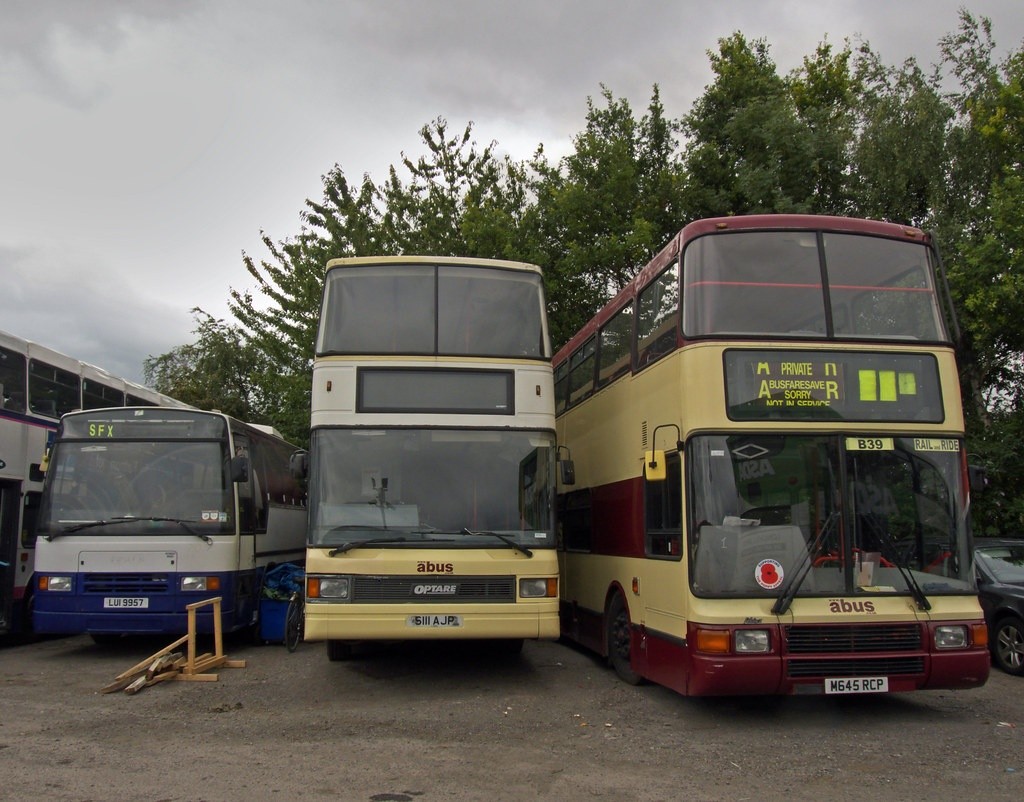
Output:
[551,213,992,705]
[24,405,311,644]
[289,256,578,667]
[1,327,285,643]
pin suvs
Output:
[884,534,1024,678]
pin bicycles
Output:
[284,565,306,653]
